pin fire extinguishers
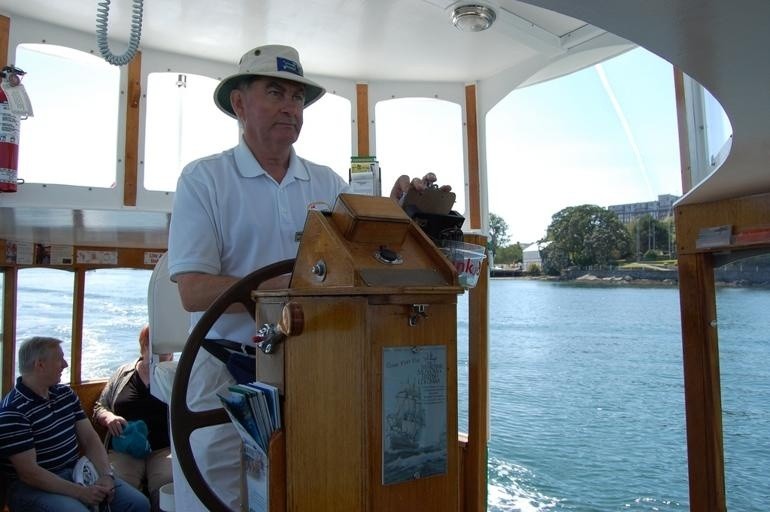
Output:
[0,64,24,192]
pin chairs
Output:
[103,430,144,492]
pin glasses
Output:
[99,484,132,511]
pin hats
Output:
[213,44,325,119]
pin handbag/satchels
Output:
[112,419,152,459]
[72,454,114,511]
[225,352,255,383]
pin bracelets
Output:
[101,472,117,481]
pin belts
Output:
[215,338,256,355]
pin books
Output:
[216,380,284,511]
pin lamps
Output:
[451,4,497,33]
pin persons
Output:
[89,324,175,512]
[166,44,455,511]
[2,336,153,512]
